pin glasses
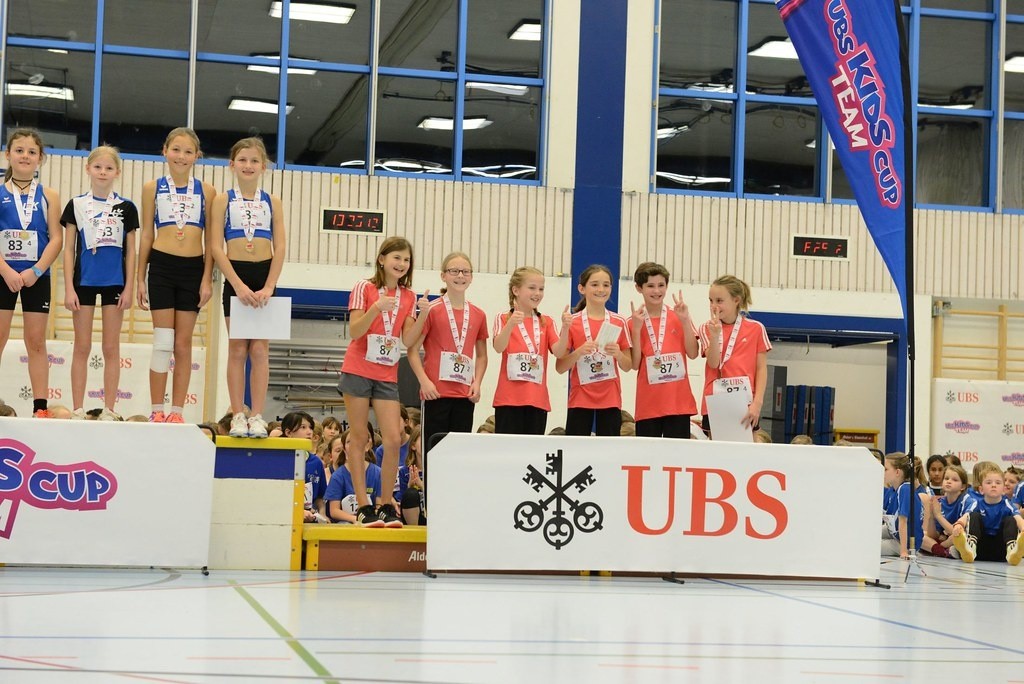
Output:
[443,268,472,276]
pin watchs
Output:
[32,266,43,277]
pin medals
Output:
[385,342,393,351]
[245,242,255,253]
[175,230,185,241]
[92,246,97,255]
[594,363,603,372]
[530,360,538,370]
[456,355,462,364]
[654,359,661,369]
[717,370,722,379]
[20,230,30,241]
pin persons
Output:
[493,267,573,436]
[212,137,285,439]
[136,127,217,422]
[556,264,633,436]
[699,276,772,443]
[0,126,62,418]
[478,411,858,447]
[337,237,430,529]
[203,405,427,528]
[626,261,699,438]
[1,399,233,436]
[406,252,488,517]
[870,448,1024,566]
[60,142,140,420]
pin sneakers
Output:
[356,505,385,528]
[952,530,977,563]
[1005,532,1024,566]
[32,409,50,419]
[69,408,88,420]
[149,410,165,423]
[164,413,185,423]
[248,414,269,438]
[377,504,403,528]
[96,408,119,421]
[229,413,248,438]
[945,545,960,559]
